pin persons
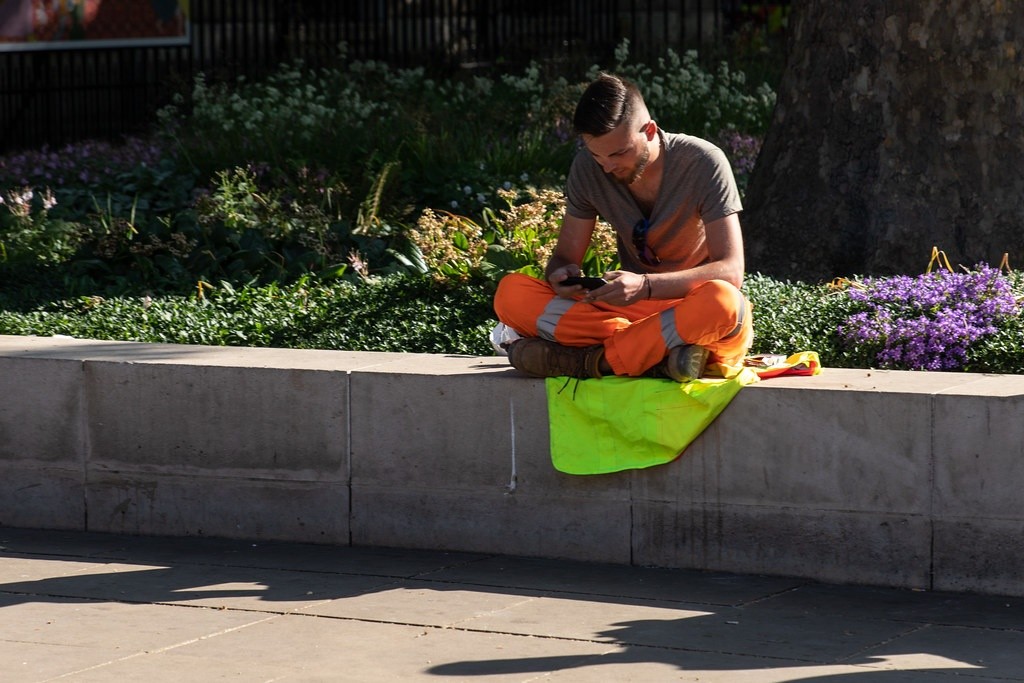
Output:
[494,72,754,382]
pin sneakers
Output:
[508,335,604,401]
[645,344,710,382]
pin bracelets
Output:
[643,275,651,300]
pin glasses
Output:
[632,218,661,267]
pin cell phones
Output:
[560,276,607,291]
